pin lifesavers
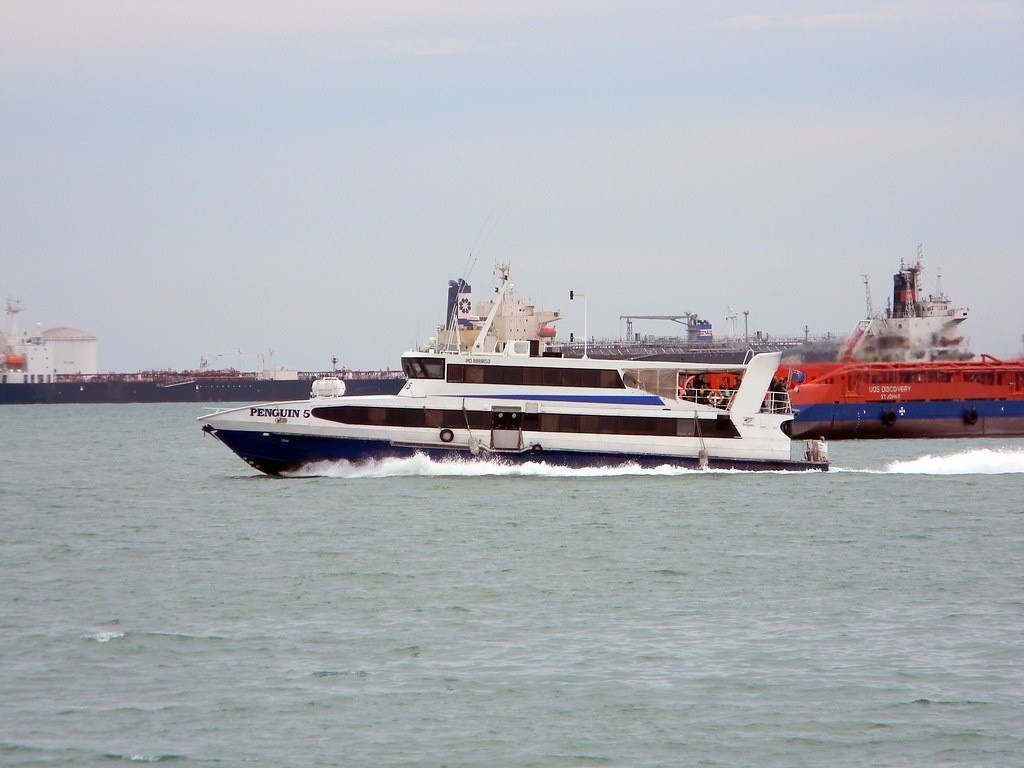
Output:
[880,410,897,427]
[964,407,979,426]
[440,428,454,443]
[706,389,724,406]
[531,444,543,455]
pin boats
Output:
[197,261,833,477]
[671,244,1024,434]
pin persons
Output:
[686,375,712,397]
[770,376,791,414]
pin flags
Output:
[791,368,807,384]
